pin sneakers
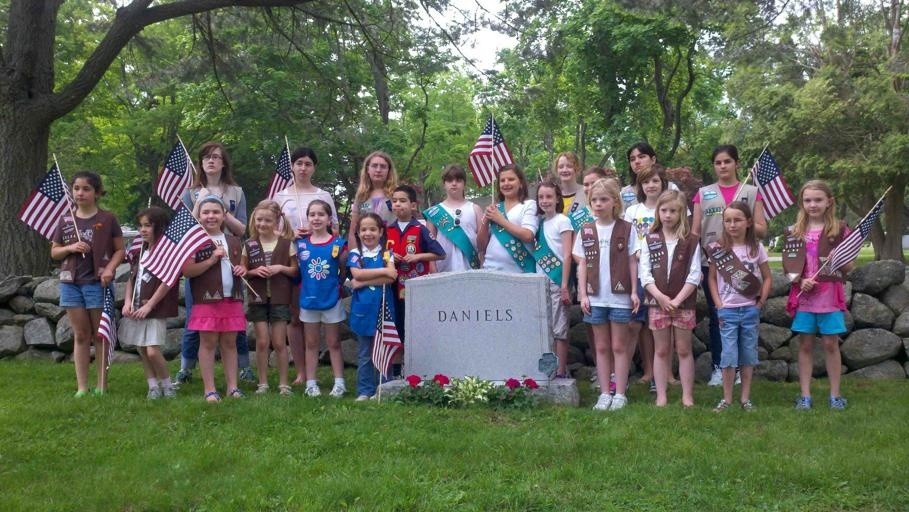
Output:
[707,364,725,388]
[588,368,687,393]
[226,387,243,400]
[732,366,744,387]
[171,373,193,388]
[255,384,272,397]
[829,395,847,410]
[239,367,258,383]
[329,383,347,398]
[354,392,378,402]
[145,385,177,402]
[290,376,306,388]
[592,393,611,411]
[795,396,812,412]
[203,390,222,405]
[279,385,295,400]
[610,394,628,412]
[72,387,110,402]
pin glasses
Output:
[454,208,462,227]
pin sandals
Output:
[741,400,757,414]
[712,399,730,414]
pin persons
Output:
[174,139,262,383]
[51,170,126,399]
[640,189,701,407]
[293,200,349,396]
[347,149,400,250]
[181,194,246,402]
[572,178,642,410]
[240,198,300,395]
[688,143,769,388]
[273,145,340,385]
[782,179,857,411]
[422,165,484,275]
[346,211,400,402]
[475,164,544,272]
[118,206,177,400]
[535,145,691,392]
[707,201,773,414]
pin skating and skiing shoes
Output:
[305,384,322,399]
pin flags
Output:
[829,194,889,274]
[19,161,77,242]
[371,289,403,380]
[154,141,195,211]
[748,147,795,218]
[466,114,516,188]
[140,205,213,288]
[265,144,294,199]
[126,230,143,265]
[97,286,119,371]
